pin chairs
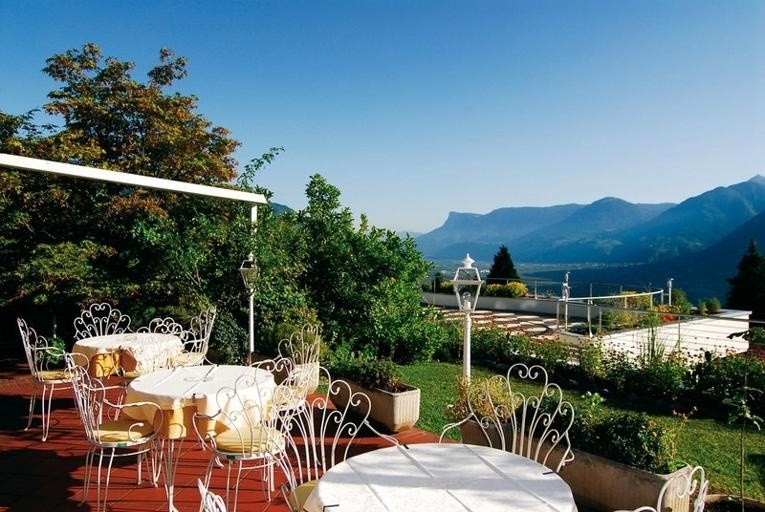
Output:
[264,362,708,511]
[16,301,321,512]
